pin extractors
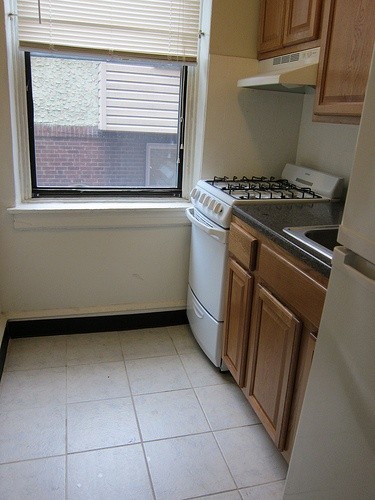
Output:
[235,46,321,95]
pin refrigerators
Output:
[282,41,374,499]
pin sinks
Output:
[282,221,342,260]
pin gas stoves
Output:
[189,161,344,205]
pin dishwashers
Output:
[184,204,232,373]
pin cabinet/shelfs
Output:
[257,0,331,62]
[222,215,331,467]
[312,0,375,126]
